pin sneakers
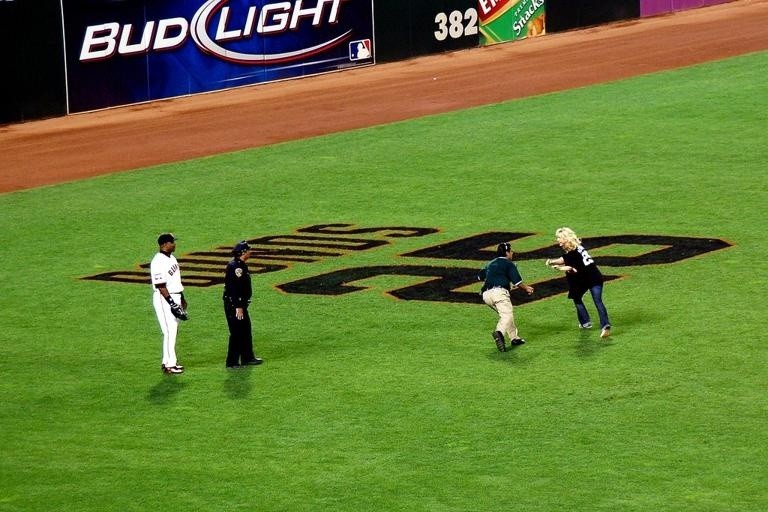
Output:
[600,324,611,337]
[579,322,592,328]
[162,364,184,374]
[226,358,263,369]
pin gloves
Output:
[165,292,190,321]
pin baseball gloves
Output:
[171,304,189,320]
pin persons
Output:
[222,240,262,368]
[544,226,611,339]
[475,241,534,351]
[150,233,189,374]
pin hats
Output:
[158,233,178,246]
[231,240,251,257]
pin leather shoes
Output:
[511,339,525,346]
[492,331,506,352]
